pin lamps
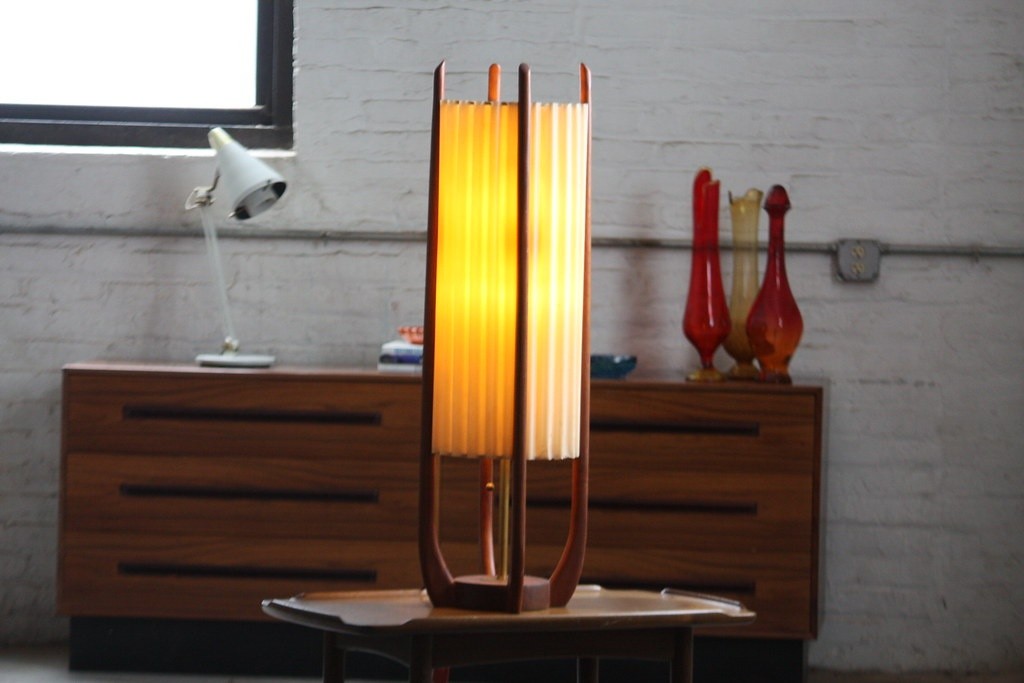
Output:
[183,127,287,369]
[417,59,593,612]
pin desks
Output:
[260,583,756,683]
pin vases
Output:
[681,170,802,384]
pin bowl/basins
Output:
[591,354,637,378]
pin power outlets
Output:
[835,239,881,282]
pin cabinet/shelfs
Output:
[52,363,826,683]
